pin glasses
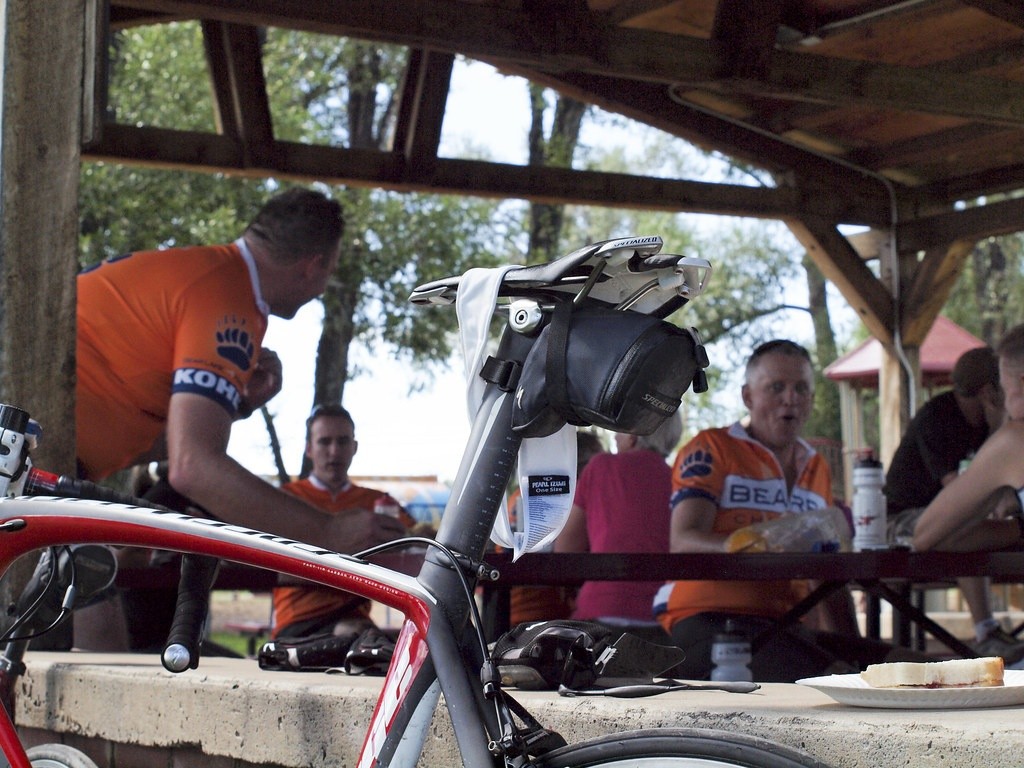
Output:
[558,633,762,697]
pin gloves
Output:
[258,632,358,671]
[344,628,397,675]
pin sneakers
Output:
[979,627,1024,665]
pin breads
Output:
[726,529,770,552]
[861,657,1006,688]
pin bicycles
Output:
[1,236,830,768]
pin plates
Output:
[796,669,1024,709]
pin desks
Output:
[114,552,1024,669]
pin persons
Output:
[476,410,682,645]
[652,339,939,682]
[884,323,1024,665]
[273,402,435,641]
[76,185,405,652]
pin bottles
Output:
[848,446,890,553]
[0,402,30,497]
[709,618,754,685]
